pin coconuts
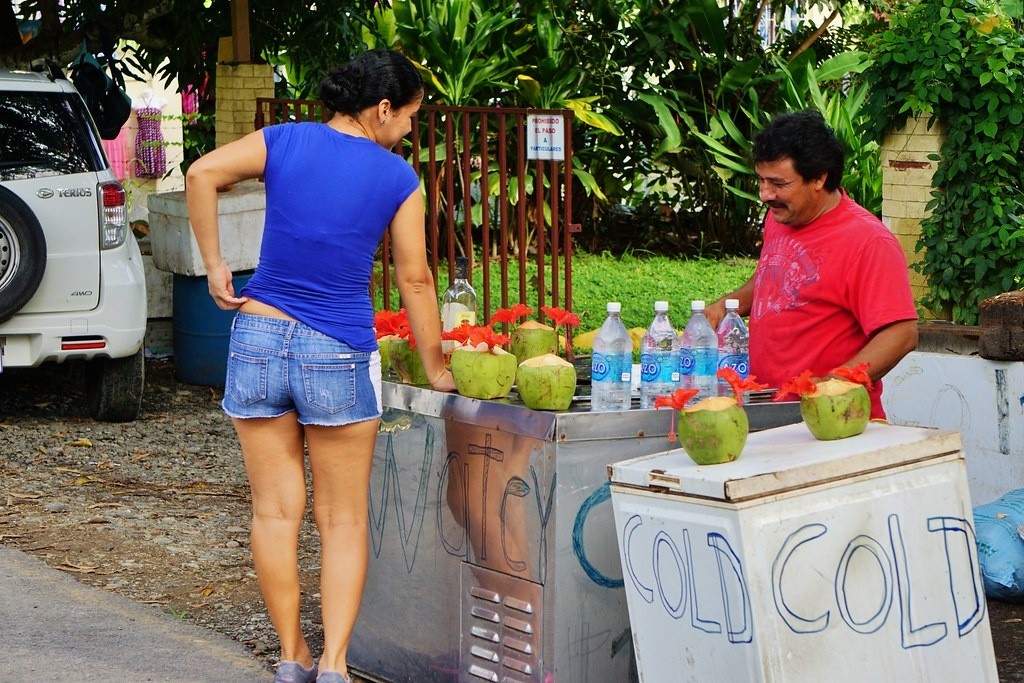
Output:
[376,319,871,466]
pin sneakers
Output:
[275,661,318,683]
[316,672,354,683]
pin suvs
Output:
[1,67,148,426]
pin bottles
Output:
[441,257,478,355]
[640,301,681,409]
[681,300,718,409]
[716,299,749,404]
[591,301,633,412]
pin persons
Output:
[699,109,920,422]
[130,87,167,177]
[430,418,553,683]
[185,48,459,683]
